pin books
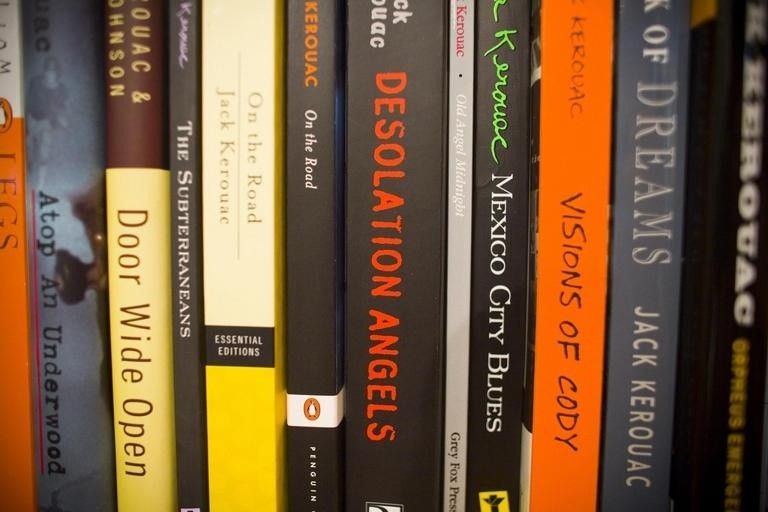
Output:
[0,1,768,512]
